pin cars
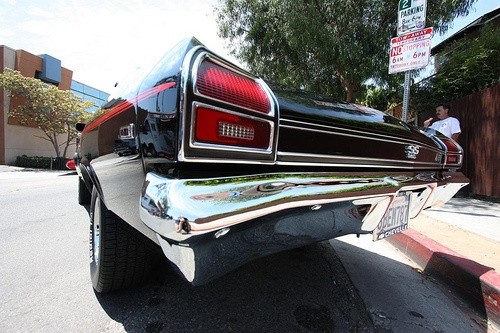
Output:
[63,36,470,294]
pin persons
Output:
[423,103,462,143]
